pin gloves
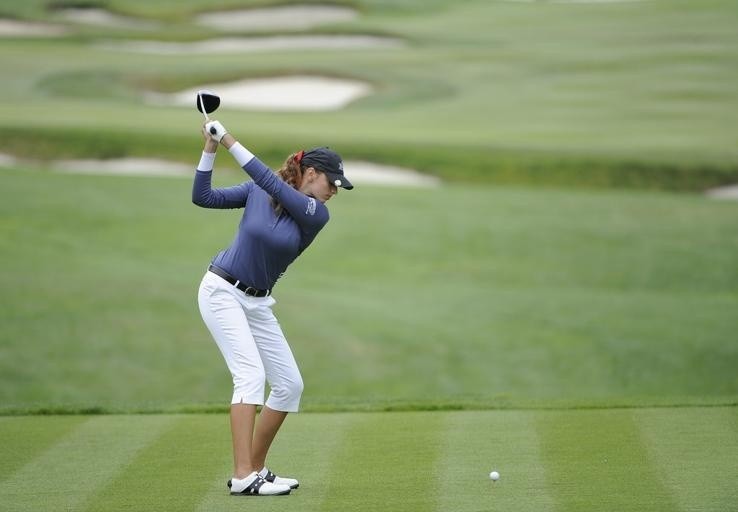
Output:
[205,118,228,142]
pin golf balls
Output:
[490,471,500,480]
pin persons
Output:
[191,119,356,497]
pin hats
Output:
[300,148,353,191]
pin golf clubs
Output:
[196,89,220,135]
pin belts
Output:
[209,264,272,297]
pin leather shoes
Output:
[231,466,299,496]
